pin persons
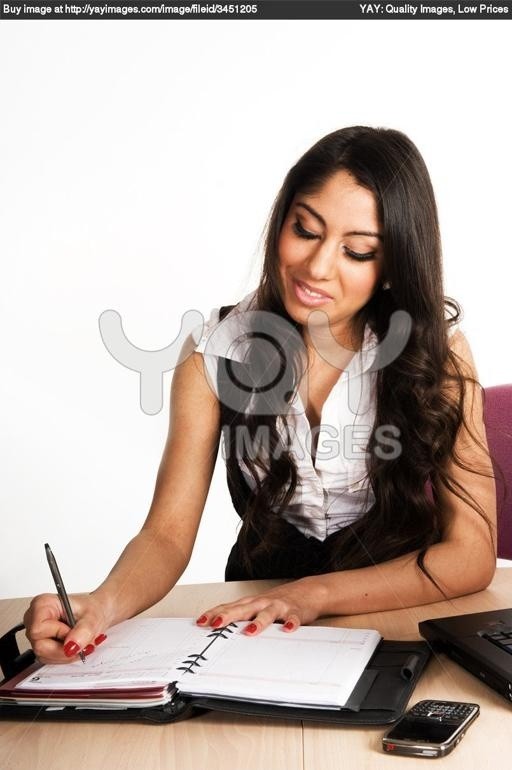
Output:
[23,126,497,666]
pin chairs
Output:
[430,386,512,556]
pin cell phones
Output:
[381,698,480,759]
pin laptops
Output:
[417,607,512,703]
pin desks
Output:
[0,562,512,760]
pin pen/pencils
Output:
[44,543,85,664]
